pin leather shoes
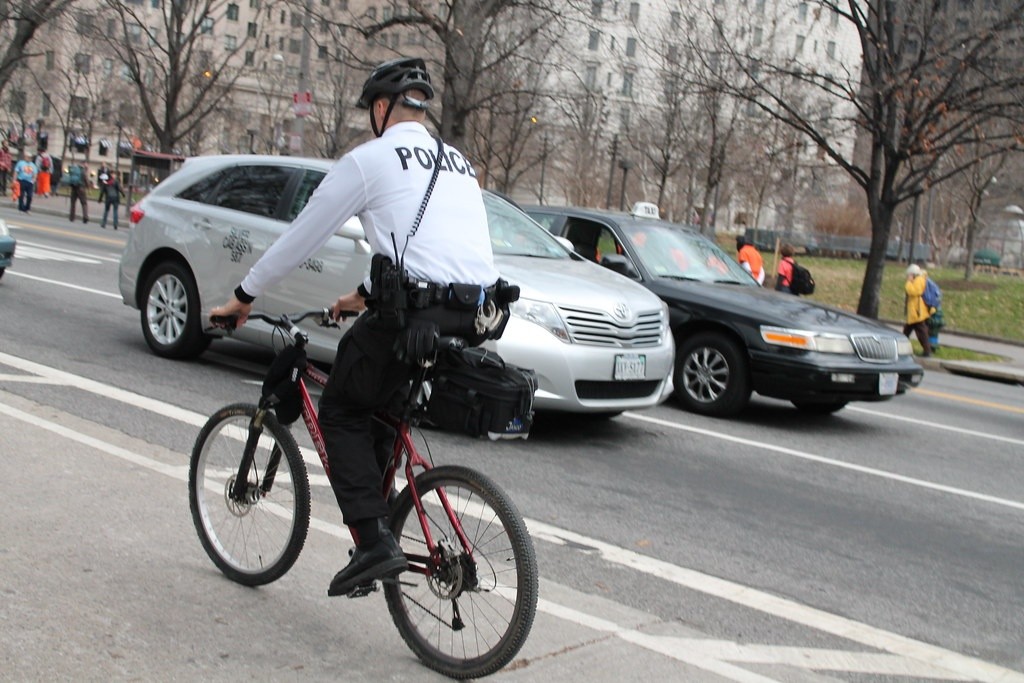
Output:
[328,478,409,595]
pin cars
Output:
[513,201,924,417]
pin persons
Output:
[736,236,766,286]
[0,140,125,229]
[775,245,796,295]
[904,263,936,356]
[209,57,495,598]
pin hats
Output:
[906,263,922,275]
[737,235,753,244]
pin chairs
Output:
[570,222,599,264]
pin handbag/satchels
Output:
[427,342,538,440]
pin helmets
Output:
[355,57,434,108]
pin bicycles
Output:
[187,309,539,680]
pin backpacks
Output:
[68,164,82,186]
[782,257,815,295]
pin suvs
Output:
[118,153,675,419]
